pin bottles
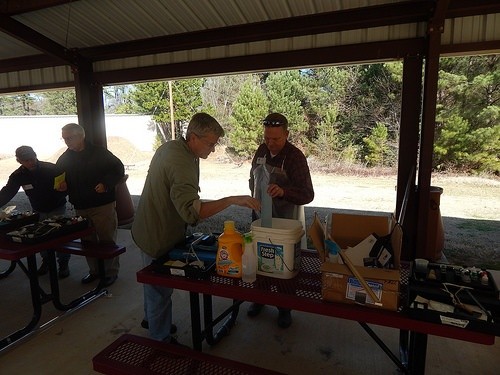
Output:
[459,266,488,285]
[241,236,256,283]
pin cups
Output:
[415,259,428,280]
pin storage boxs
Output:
[309,210,400,312]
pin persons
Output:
[131,113,261,351]
[0,145,72,278]
[249,113,314,329]
[49,122,124,288]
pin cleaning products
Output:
[324,239,342,264]
[216,221,245,277]
[242,233,256,284]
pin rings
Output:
[276,187,279,190]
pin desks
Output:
[0,214,109,353]
[136,232,496,374]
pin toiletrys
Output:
[65,195,76,218]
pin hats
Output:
[15,146,37,161]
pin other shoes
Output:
[279,310,291,328]
[247,304,265,317]
[141,319,192,350]
[37,257,48,275]
[57,257,70,278]
[81,273,118,287]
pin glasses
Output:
[258,120,285,127]
[192,131,219,148]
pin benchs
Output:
[58,242,126,291]
[93,334,286,375]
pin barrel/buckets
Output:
[251,217,303,279]
